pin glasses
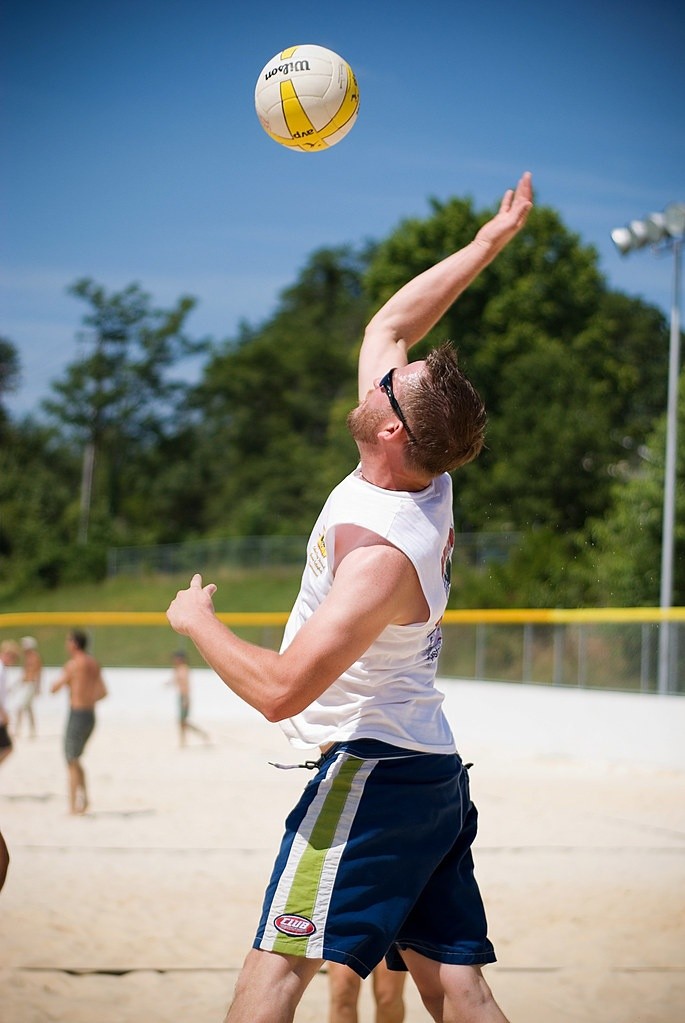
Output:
[379,370,418,449]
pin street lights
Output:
[610,202,685,694]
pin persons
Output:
[171,650,209,744]
[51,627,107,814]
[0,639,20,762]
[8,635,42,738]
[165,172,533,1023]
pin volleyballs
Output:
[253,43,361,154]
